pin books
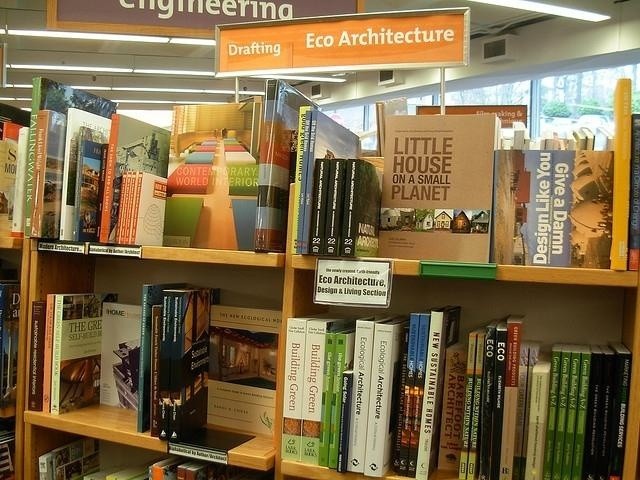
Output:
[291,101,638,272]
[0,264,283,479]
[284,306,632,479]
[0,78,320,253]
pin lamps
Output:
[472,0,611,23]
